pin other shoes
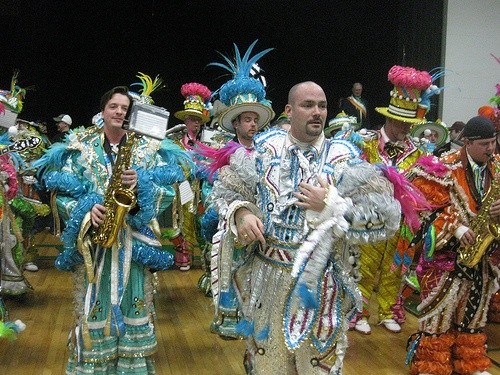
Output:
[355,318,370,334]
[24,262,38,271]
[382,318,402,332]
[180,264,190,270]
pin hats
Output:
[328,112,357,126]
[375,65,453,123]
[447,121,466,131]
[53,114,72,125]
[173,82,211,122]
[463,116,498,139]
[410,118,448,146]
[325,121,351,137]
[207,39,275,134]
[0,70,25,128]
[278,111,289,121]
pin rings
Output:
[243,234,248,239]
[301,195,308,202]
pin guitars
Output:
[0,134,42,155]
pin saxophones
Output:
[90,131,137,248]
[456,153,500,268]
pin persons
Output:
[30,86,185,375]
[165,77,292,340]
[0,90,47,339]
[53,114,72,233]
[342,83,371,132]
[324,91,465,333]
[208,81,402,375]
[400,116,500,375]
